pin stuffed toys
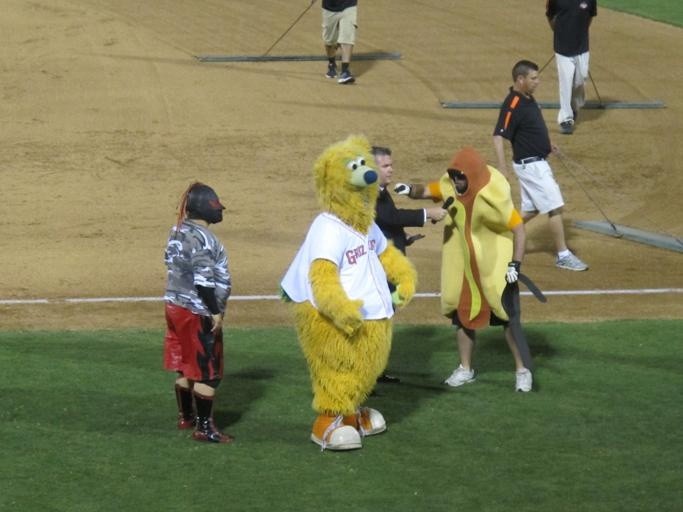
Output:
[281,134,418,451]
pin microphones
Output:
[432,197,454,224]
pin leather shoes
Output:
[378,373,399,382]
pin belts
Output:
[516,156,541,164]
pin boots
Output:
[192,390,234,443]
[174,384,196,429]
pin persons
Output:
[368,146,448,384]
[320,1,358,83]
[494,59,590,271]
[545,1,597,133]
[395,148,548,392]
[164,182,235,443]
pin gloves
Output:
[394,183,410,195]
[506,260,520,283]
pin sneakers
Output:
[561,122,572,134]
[445,365,476,387]
[516,369,532,392]
[338,72,354,84]
[556,254,588,271]
[326,64,337,78]
